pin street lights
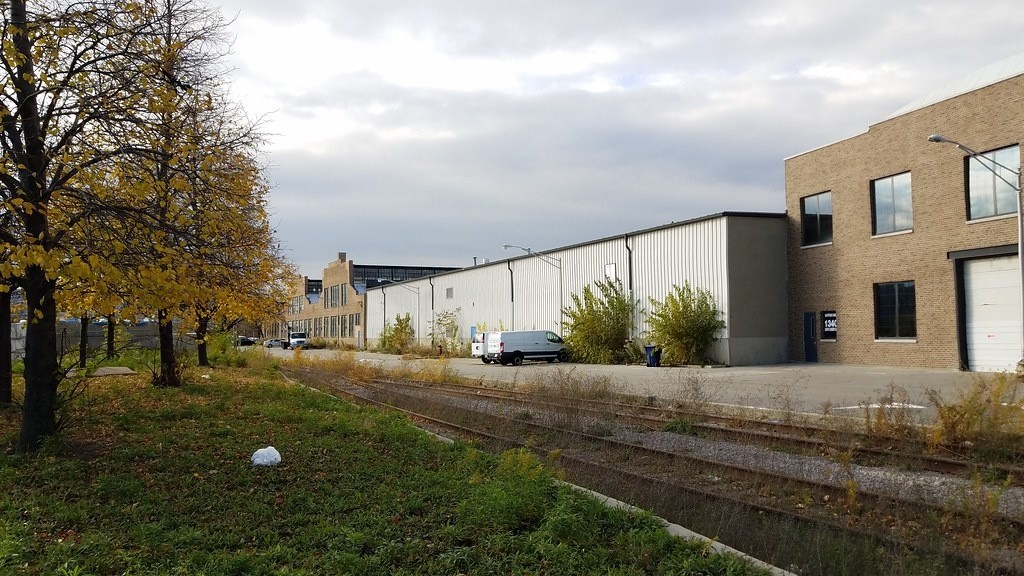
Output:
[378,278,420,344]
[927,133,1024,359]
[503,245,562,339]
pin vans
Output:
[488,330,572,366]
[472,332,500,364]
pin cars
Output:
[237,332,308,350]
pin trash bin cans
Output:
[644,344,662,367]
